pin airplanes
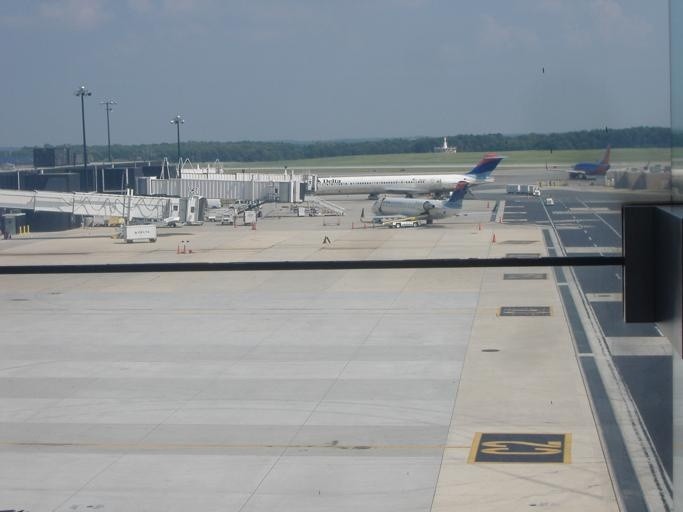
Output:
[360,180,469,224]
[544,144,650,181]
[304,153,504,201]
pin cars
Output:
[219,200,250,225]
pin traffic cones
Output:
[176,244,192,254]
[490,233,496,243]
[350,222,375,229]
[486,202,489,208]
[251,222,256,230]
[478,223,482,231]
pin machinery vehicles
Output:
[381,212,429,227]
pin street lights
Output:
[169,112,184,162]
[99,95,117,162]
[73,84,93,167]
